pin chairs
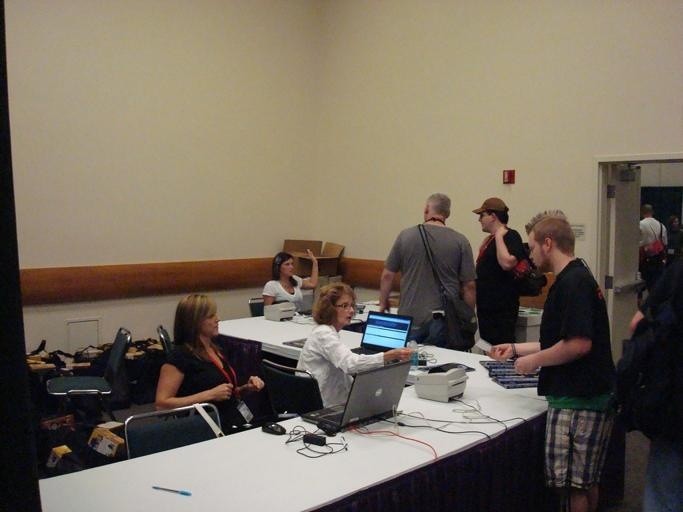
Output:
[157,326,176,358]
[45,326,133,422]
[249,298,267,319]
[124,402,222,457]
[261,359,323,419]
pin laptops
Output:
[301,361,411,431]
[351,310,414,356]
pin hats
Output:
[471,198,508,214]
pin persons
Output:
[300,281,411,408]
[472,198,530,354]
[605,200,683,512]
[155,294,265,437]
[380,193,479,352]
[262,248,319,314]
[486,216,616,511]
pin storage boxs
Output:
[46,445,72,470]
[282,240,344,278]
[296,278,344,312]
[87,420,123,460]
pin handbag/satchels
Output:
[442,294,477,350]
[617,261,680,441]
[513,257,547,297]
[639,240,667,265]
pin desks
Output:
[27,300,557,511]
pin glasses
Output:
[333,302,354,309]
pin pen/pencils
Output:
[153,487,191,495]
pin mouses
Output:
[262,422,287,435]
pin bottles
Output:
[408,336,418,371]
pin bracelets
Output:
[511,343,518,356]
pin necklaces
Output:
[425,216,446,225]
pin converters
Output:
[303,433,327,447]
